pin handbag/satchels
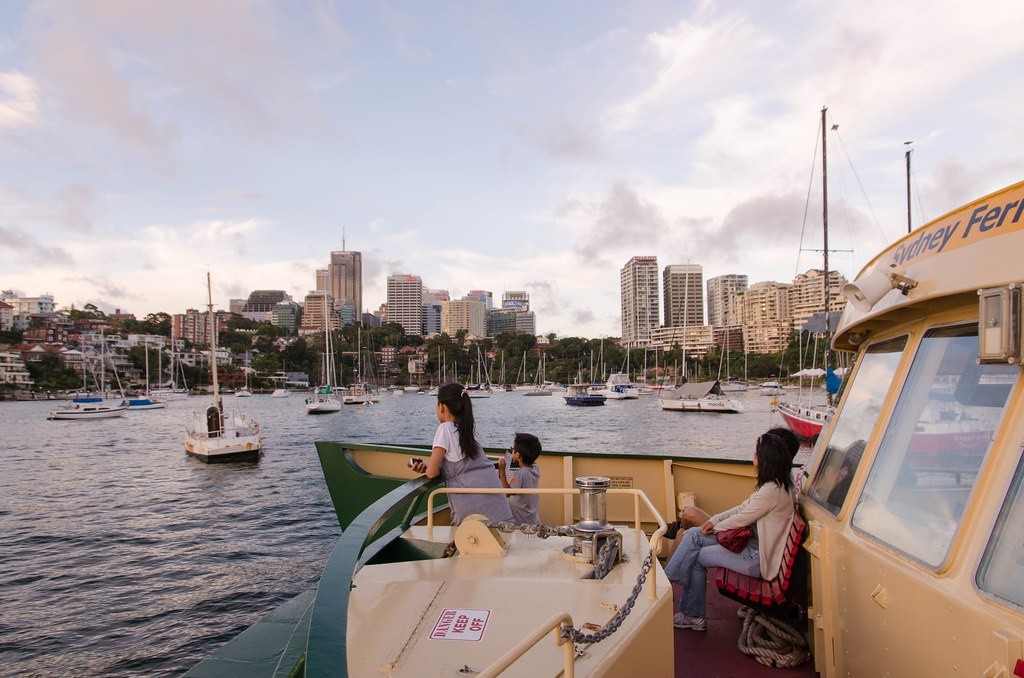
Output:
[716,526,754,553]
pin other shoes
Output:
[673,609,708,631]
[657,523,677,539]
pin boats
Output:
[759,380,786,397]
[416,388,425,394]
[388,385,398,389]
[588,372,640,400]
[563,377,610,406]
[392,390,404,398]
[253,370,311,393]
[235,349,253,397]
[404,383,420,391]
[378,386,388,392]
[503,383,517,393]
[748,378,760,392]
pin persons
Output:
[498,432,544,526]
[662,426,801,633]
[406,382,515,526]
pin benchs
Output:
[715,507,808,633]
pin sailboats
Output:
[461,346,493,399]
[541,352,566,392]
[45,336,127,419]
[116,338,167,411]
[656,256,743,413]
[645,345,675,391]
[429,349,446,397]
[429,343,444,389]
[271,359,290,398]
[185,272,263,466]
[516,351,534,390]
[772,105,994,461]
[314,353,333,393]
[305,274,341,413]
[522,359,552,397]
[717,313,747,393]
[342,327,380,404]
[150,314,191,400]
[619,341,655,395]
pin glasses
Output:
[758,432,767,447]
